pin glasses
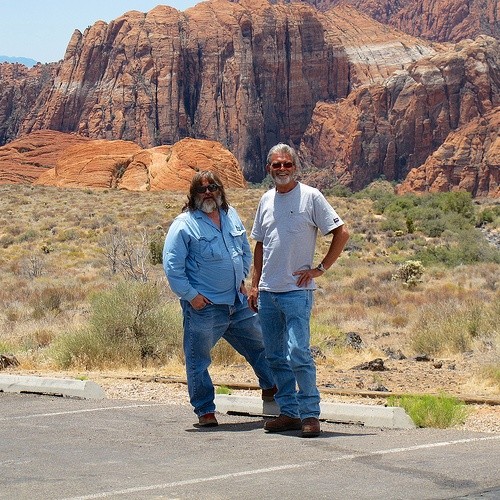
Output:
[268,162,296,169]
[196,183,219,193]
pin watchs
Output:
[314,262,334,280]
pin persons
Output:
[246,142,349,438]
[160,169,280,427]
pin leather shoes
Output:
[199,413,217,426]
[264,413,301,432]
[301,417,322,436]
[261,386,278,400]
